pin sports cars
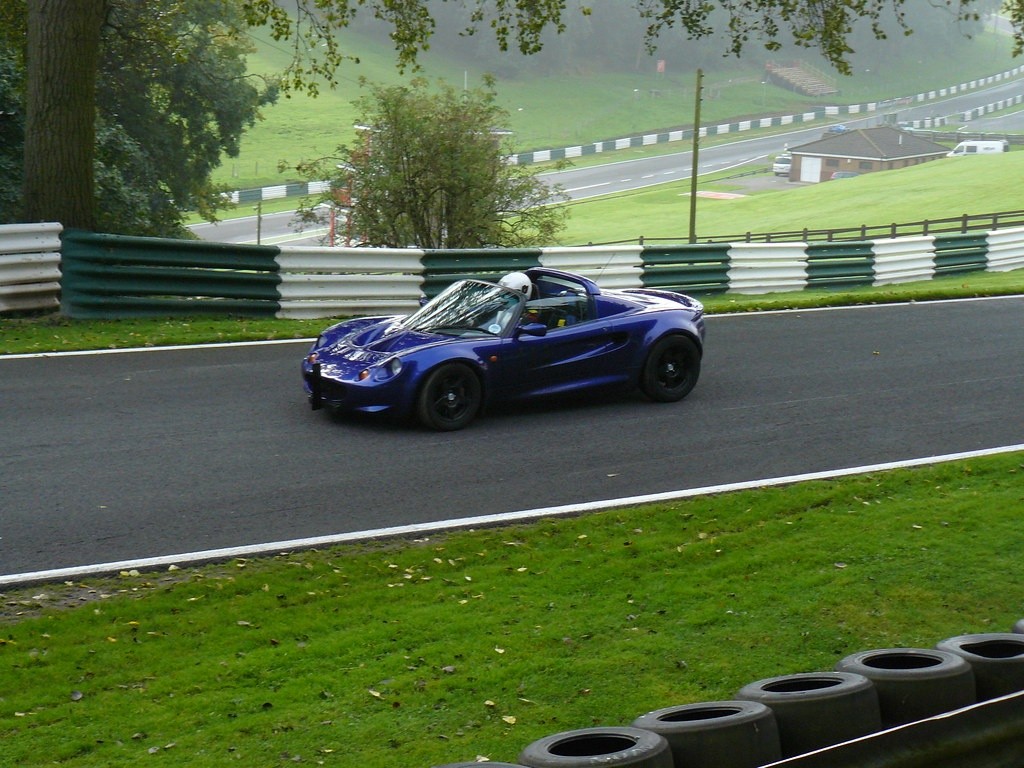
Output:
[300,265,706,433]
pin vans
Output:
[773,154,792,177]
[947,140,1010,160]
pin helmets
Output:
[497,272,533,309]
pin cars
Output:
[829,124,850,134]
[829,171,858,180]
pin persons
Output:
[463,271,539,336]
